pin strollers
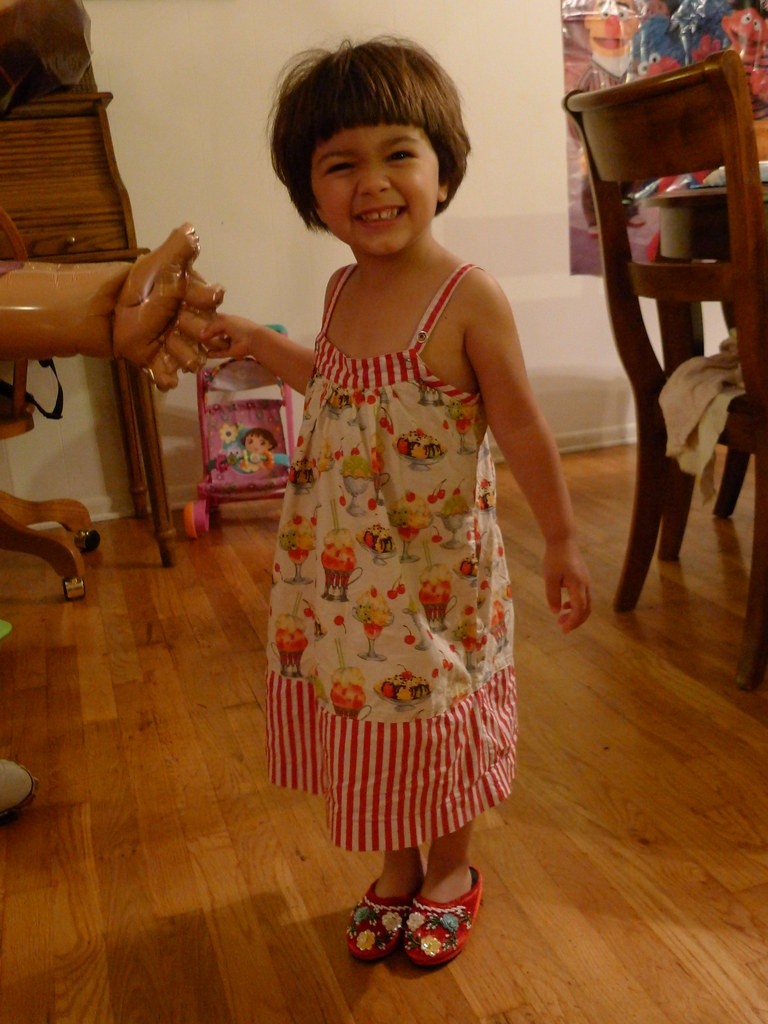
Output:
[183,324,296,538]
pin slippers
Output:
[404,864,483,965]
[345,879,414,962]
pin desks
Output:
[0,89,175,570]
[639,183,768,559]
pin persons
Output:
[197,36,595,969]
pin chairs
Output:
[562,50,768,691]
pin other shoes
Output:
[0,758,39,817]
